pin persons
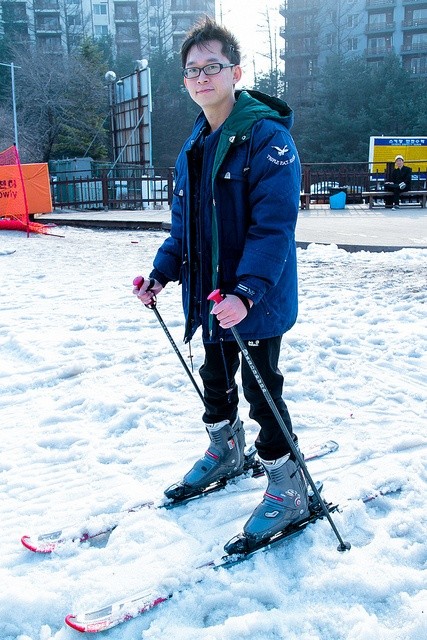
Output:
[384,155,412,211]
[132,16,312,540]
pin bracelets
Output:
[233,291,250,314]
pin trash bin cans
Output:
[328,187,347,209]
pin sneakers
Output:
[183,411,246,489]
[244,434,311,540]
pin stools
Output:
[300,192,310,210]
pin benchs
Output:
[361,191,425,210]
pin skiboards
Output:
[20,440,401,633]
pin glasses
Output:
[182,63,236,79]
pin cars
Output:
[155,175,169,192]
